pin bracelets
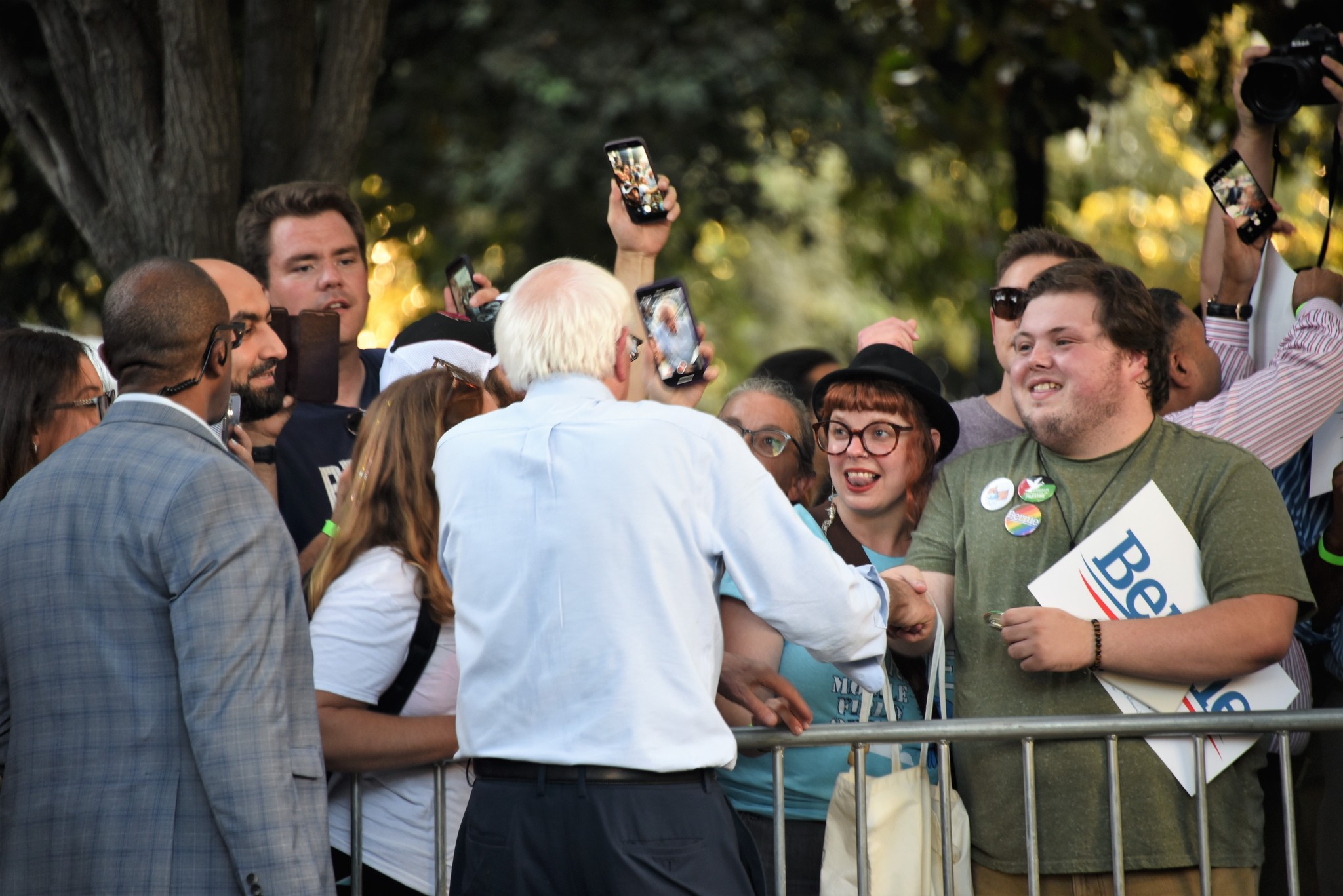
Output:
[1206,297,1254,322]
[321,520,342,541]
[1293,297,1317,320]
[1090,619,1105,670]
[1318,534,1342,567]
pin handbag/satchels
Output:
[820,767,977,896]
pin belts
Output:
[468,757,720,785]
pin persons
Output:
[753,348,841,423]
[613,160,666,215]
[1146,45,1343,895]
[717,343,960,895]
[1,254,339,896]
[188,257,286,424]
[648,293,700,374]
[712,376,819,500]
[229,179,500,593]
[1,327,254,499]
[429,256,937,896]
[856,230,1105,469]
[879,260,1317,896]
[307,366,498,895]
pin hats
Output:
[812,342,961,464]
[378,291,509,393]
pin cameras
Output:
[1239,0,1343,122]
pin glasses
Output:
[429,356,481,417]
[721,420,807,466]
[627,333,643,362]
[989,286,1030,320]
[203,322,246,363]
[810,420,912,458]
[44,389,116,422]
[345,409,366,435]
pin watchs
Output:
[251,444,278,465]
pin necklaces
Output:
[1039,413,1156,558]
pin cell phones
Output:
[604,136,669,224]
[221,393,242,452]
[269,307,288,397]
[444,254,486,322]
[1204,148,1278,245]
[295,310,340,402]
[636,277,707,387]
